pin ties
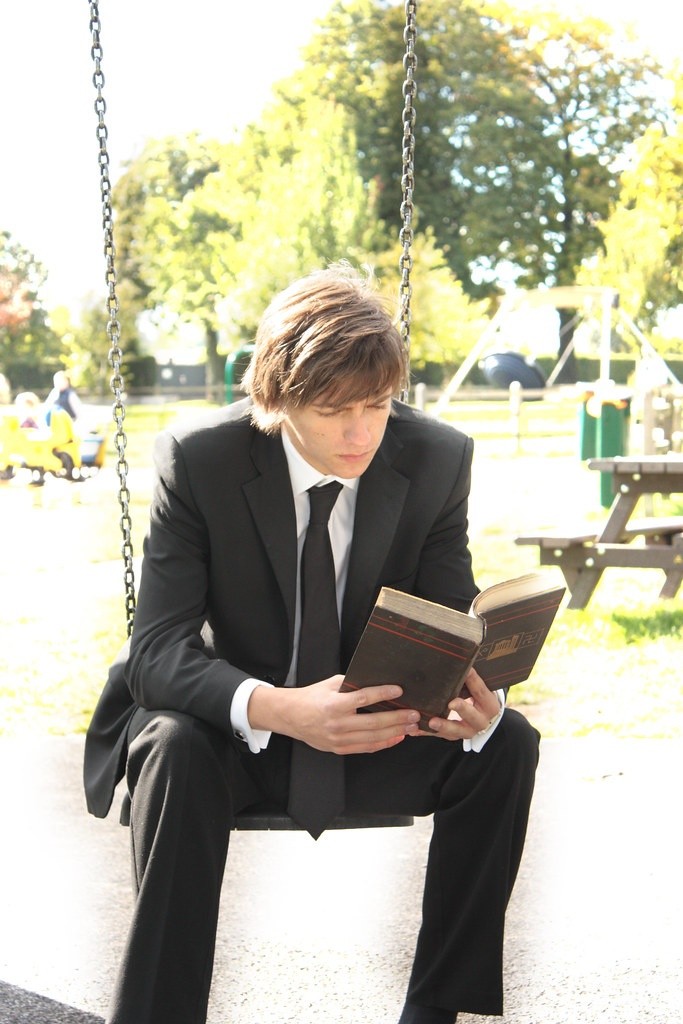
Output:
[288,481,346,841]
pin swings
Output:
[89,0,417,832]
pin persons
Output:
[43,370,91,447]
[80,271,541,1024]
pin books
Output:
[337,563,567,733]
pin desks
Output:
[540,452,683,610]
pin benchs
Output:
[513,516,683,549]
